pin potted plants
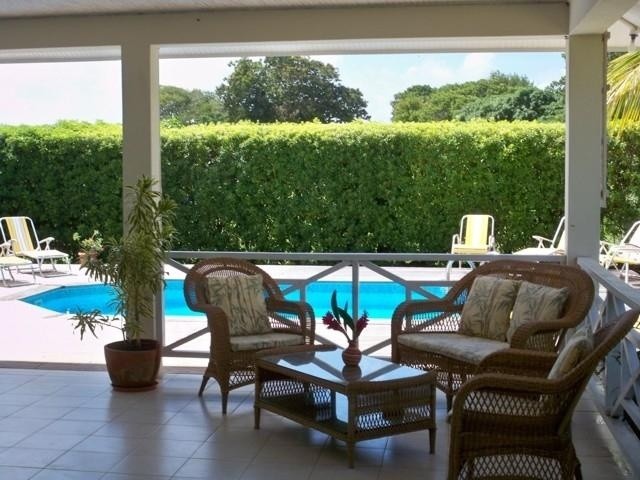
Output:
[68,173,180,390]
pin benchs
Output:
[388,257,594,411]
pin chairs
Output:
[184,257,316,416]
[511,214,565,258]
[446,214,498,276]
[599,220,640,286]
[448,307,640,479]
[1,215,68,288]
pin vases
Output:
[342,341,362,366]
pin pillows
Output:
[539,324,595,408]
[203,272,273,337]
[456,274,569,351]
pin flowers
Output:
[322,289,370,340]
